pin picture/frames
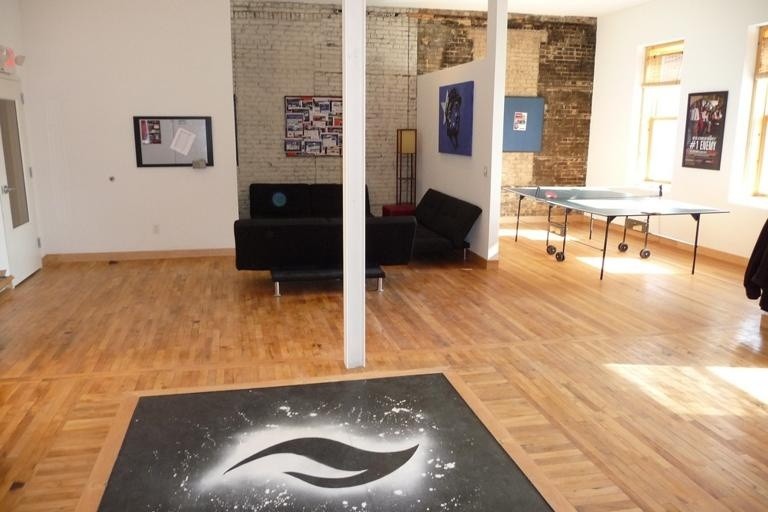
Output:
[503,95,544,155]
[683,90,729,171]
[283,95,342,158]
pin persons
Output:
[690,102,725,137]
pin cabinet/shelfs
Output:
[383,202,416,217]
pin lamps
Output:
[396,129,417,204]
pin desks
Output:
[511,184,731,282]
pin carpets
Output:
[97,372,557,512]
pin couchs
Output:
[410,188,482,264]
[234,215,418,298]
[248,183,375,218]
